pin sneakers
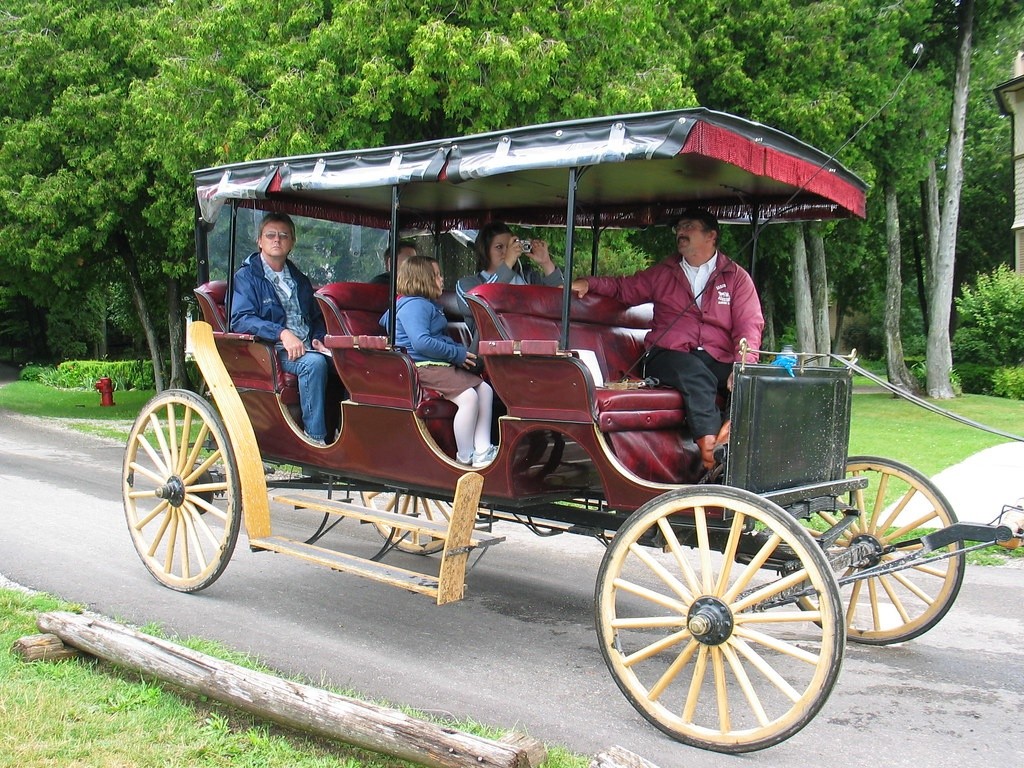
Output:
[472,444,498,467]
[456,451,473,464]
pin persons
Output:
[230,212,350,446]
[369,239,417,284]
[377,256,500,468]
[456,223,565,381]
[557,208,763,480]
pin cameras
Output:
[514,239,532,253]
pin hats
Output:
[669,207,719,228]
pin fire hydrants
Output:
[95,377,116,407]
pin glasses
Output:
[261,231,288,240]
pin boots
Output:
[695,419,730,482]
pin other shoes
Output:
[334,428,340,441]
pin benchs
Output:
[312,281,457,418]
[463,280,684,432]
[192,280,296,389]
